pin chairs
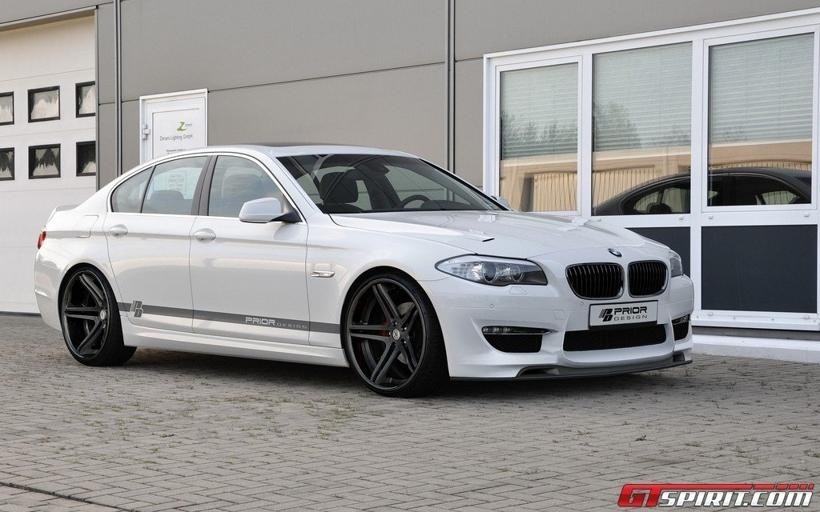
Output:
[151,190,186,215]
[320,172,366,211]
[646,203,672,214]
[223,174,264,217]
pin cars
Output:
[591,165,818,215]
[31,141,696,399]
